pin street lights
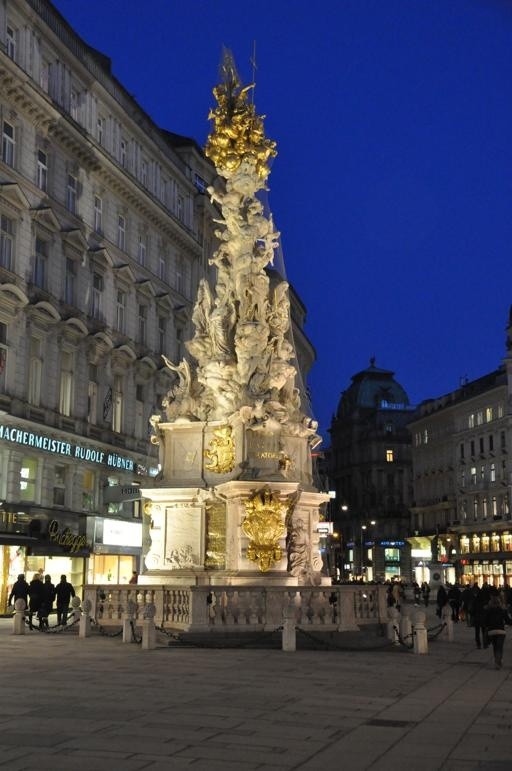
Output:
[360,523,368,576]
[327,488,338,521]
[331,531,338,582]
[340,503,349,580]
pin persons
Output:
[328,576,511,672]
[8,573,29,609]
[27,573,42,630]
[37,574,55,630]
[160,82,320,438]
[54,574,75,625]
[129,570,139,586]
